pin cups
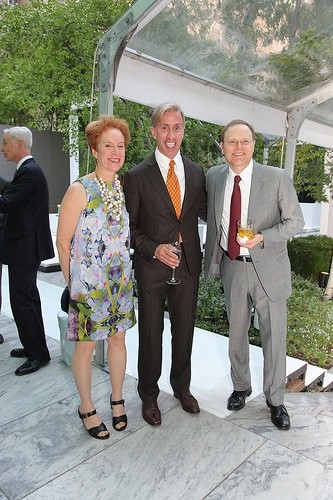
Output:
[237,219,255,240]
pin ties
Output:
[227,175,241,261]
[166,159,183,245]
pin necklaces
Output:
[95,169,123,220]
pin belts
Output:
[222,248,252,262]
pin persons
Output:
[0,126,55,376]
[206,119,304,429]
[56,114,137,440]
[123,103,207,426]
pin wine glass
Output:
[166,243,182,285]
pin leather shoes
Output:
[227,385,252,410]
[266,399,290,430]
[10,349,28,358]
[15,357,51,376]
[174,391,201,413]
[142,398,161,425]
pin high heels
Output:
[110,394,127,431]
[78,406,110,439]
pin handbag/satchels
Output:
[60,285,69,314]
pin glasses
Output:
[222,139,252,145]
